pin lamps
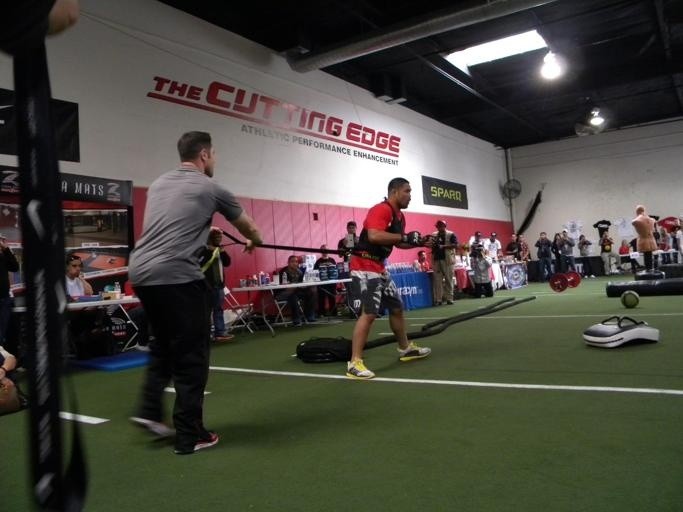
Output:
[541,52,563,79]
[590,110,605,125]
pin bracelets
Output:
[1,366,7,373]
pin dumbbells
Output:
[549,271,581,292]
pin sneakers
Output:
[346,359,375,379]
[617,267,624,272]
[433,302,442,305]
[397,342,431,362]
[293,322,301,327]
[175,433,220,454]
[128,417,176,437]
[584,276,588,278]
[212,334,234,342]
[590,275,595,277]
[448,301,453,304]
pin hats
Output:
[475,232,481,237]
[438,220,447,226]
[491,232,496,236]
[562,229,568,233]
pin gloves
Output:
[422,235,442,248]
[402,231,422,245]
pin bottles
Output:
[273,270,279,286]
[113,282,121,300]
[259,271,264,287]
[264,272,271,286]
[253,274,258,289]
[282,272,287,285]
[246,275,253,287]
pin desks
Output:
[490,264,503,293]
[456,266,494,292]
[376,271,431,314]
[501,261,528,289]
[232,278,353,337]
[12,296,140,353]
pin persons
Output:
[346,178,437,380]
[599,230,625,276]
[0,1,82,51]
[558,229,576,272]
[484,232,503,261]
[273,255,317,329]
[199,230,235,344]
[518,234,531,261]
[64,255,93,300]
[534,231,553,282]
[470,245,494,298]
[578,235,595,279]
[472,231,484,249]
[313,244,340,317]
[505,234,519,262]
[0,339,21,415]
[337,220,359,262]
[0,234,21,335]
[551,233,562,272]
[430,220,459,307]
[618,227,683,276]
[418,250,430,271]
[128,132,263,454]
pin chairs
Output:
[270,288,307,329]
[119,302,153,355]
[223,286,259,334]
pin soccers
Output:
[620,290,639,308]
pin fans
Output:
[503,179,522,200]
